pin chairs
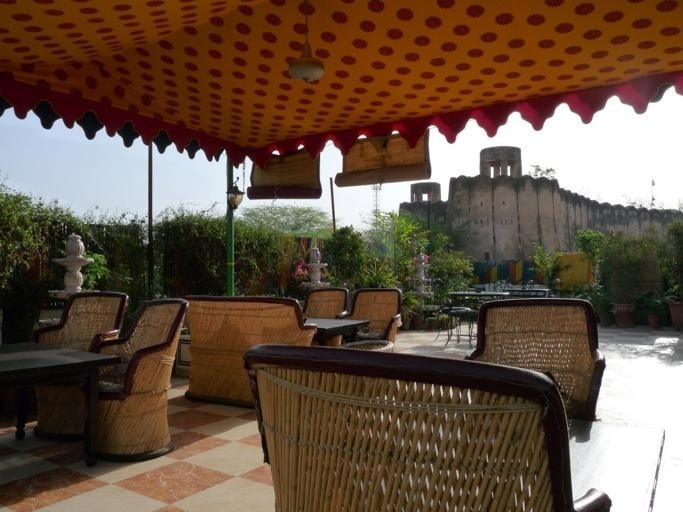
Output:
[465,301,605,418]
[83,301,191,462]
[182,297,313,406]
[242,339,612,512]
[343,288,407,346]
[298,290,342,345]
[431,280,536,345]
[30,293,128,436]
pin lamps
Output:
[286,0,324,82]
[224,176,245,210]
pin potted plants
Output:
[636,290,665,330]
[608,249,645,329]
[661,266,683,332]
[0,193,49,343]
[593,283,613,327]
